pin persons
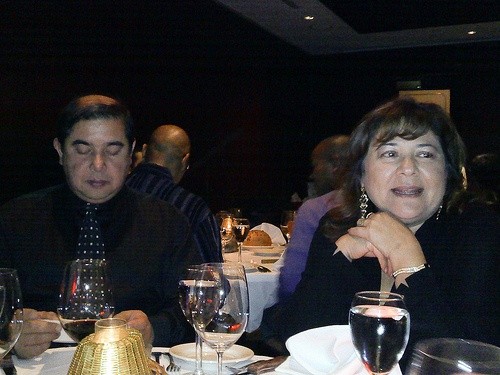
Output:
[259,135,354,340]
[0,94,207,359]
[466,154,500,204]
[127,124,230,303]
[275,97,500,370]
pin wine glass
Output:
[193,263,250,375]
[178,265,226,375]
[232,219,250,263]
[216,214,233,263]
[280,211,297,244]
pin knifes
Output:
[2,352,16,375]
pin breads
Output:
[242,230,272,246]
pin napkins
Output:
[275,323,403,375]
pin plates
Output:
[254,249,284,255]
[242,246,275,251]
[169,342,254,363]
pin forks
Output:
[163,352,181,372]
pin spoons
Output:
[226,360,263,374]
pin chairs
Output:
[250,223,286,245]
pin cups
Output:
[405,337,500,375]
[349,291,410,375]
[0,268,24,360]
[57,259,119,345]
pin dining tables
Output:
[0,347,286,375]
[223,241,290,334]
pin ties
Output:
[69,202,115,319]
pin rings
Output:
[366,212,374,218]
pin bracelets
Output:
[392,265,428,276]
